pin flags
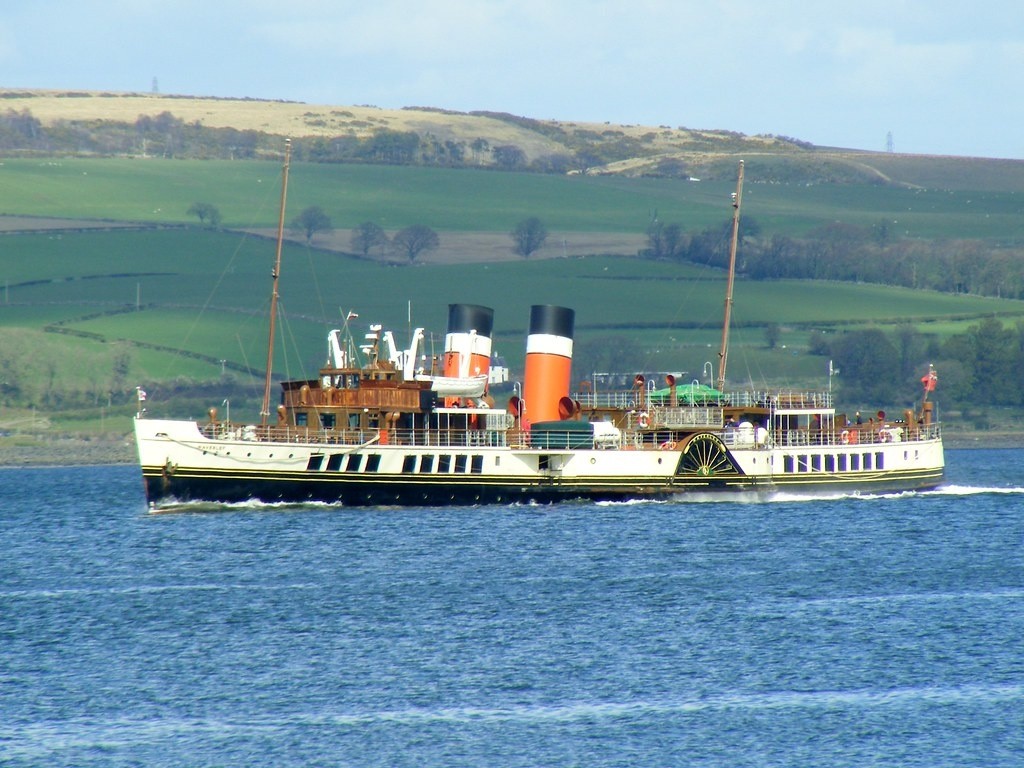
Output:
[921,370,938,391]
[137,389,146,401]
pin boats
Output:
[132,138,946,508]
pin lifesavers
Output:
[841,430,850,444]
[638,412,650,428]
[658,441,677,451]
[879,429,887,442]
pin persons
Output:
[196,422,249,442]
[854,412,862,424]
[723,418,739,429]
